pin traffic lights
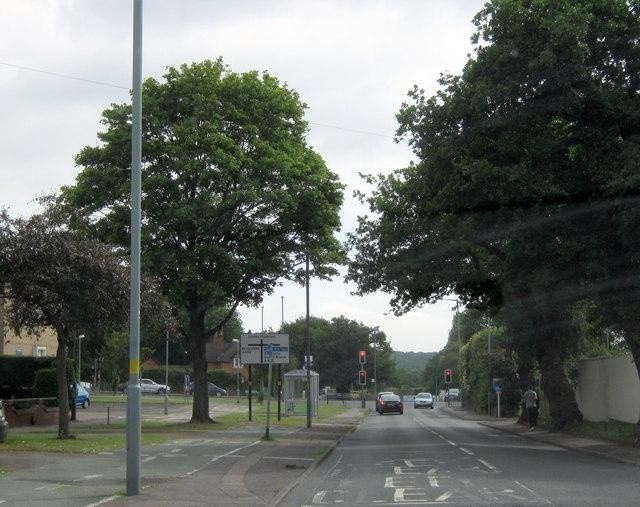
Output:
[358,351,367,386]
[445,370,451,383]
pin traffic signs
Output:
[240,333,290,364]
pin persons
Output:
[521,384,538,431]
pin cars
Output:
[118,378,171,396]
[71,384,91,408]
[376,391,404,415]
[185,380,227,398]
[445,389,461,402]
[414,393,435,409]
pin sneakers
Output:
[529,427,535,432]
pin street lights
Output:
[78,334,85,382]
